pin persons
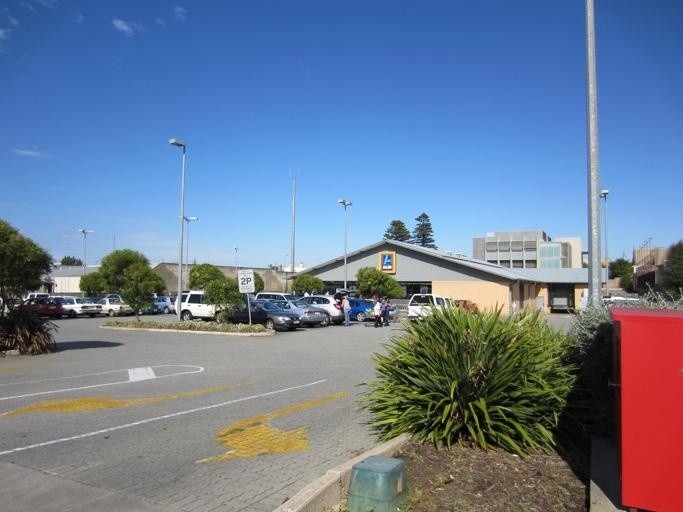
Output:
[373,296,390,328]
[340,296,352,326]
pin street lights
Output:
[337,198,352,289]
[78,229,94,298]
[599,189,610,299]
[169,138,198,323]
[235,247,238,278]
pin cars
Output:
[407,294,478,320]
